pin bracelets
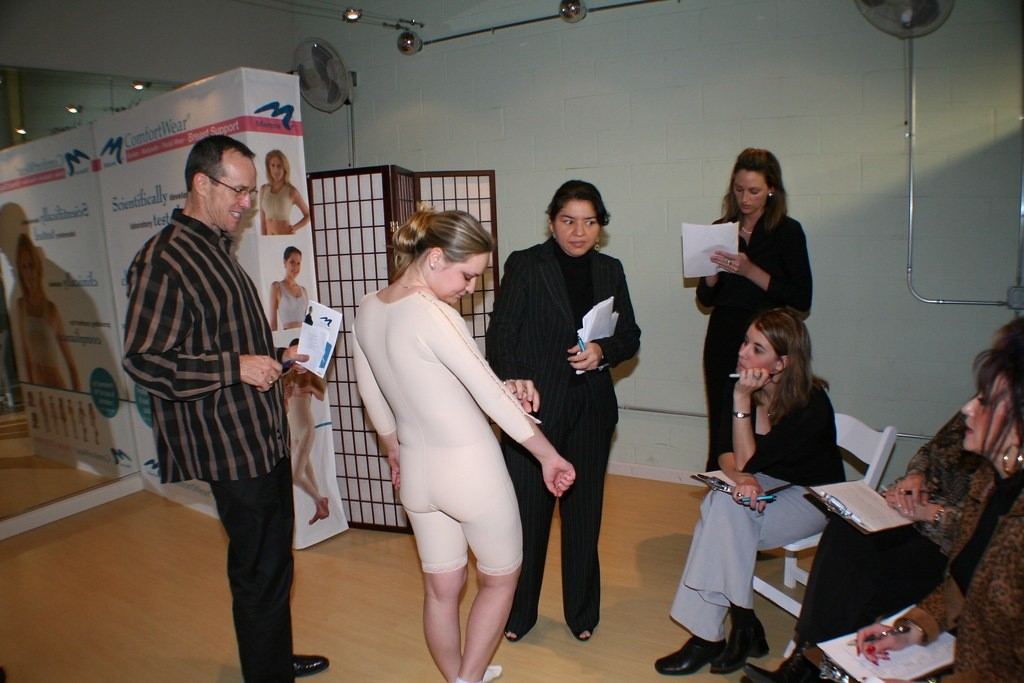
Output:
[933,505,944,528]
[906,619,923,633]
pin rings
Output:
[754,376,759,380]
[737,493,744,497]
[727,259,731,265]
[752,490,757,493]
[898,489,905,495]
[267,376,273,386]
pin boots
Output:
[744,646,821,683]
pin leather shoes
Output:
[293,654,331,678]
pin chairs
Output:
[754,414,897,660]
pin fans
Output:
[852,0,956,39]
[291,35,359,116]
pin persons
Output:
[259,149,310,235]
[688,146,815,474]
[352,203,576,683]
[283,338,330,526]
[653,307,847,676]
[747,318,1024,683]
[121,133,329,683]
[16,233,81,393]
[269,245,310,331]
[487,181,640,642]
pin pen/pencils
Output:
[900,488,942,494]
[739,495,778,501]
[577,336,585,353]
[846,625,911,646]
[282,359,295,373]
[729,373,741,377]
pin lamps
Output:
[66,106,82,113]
[345,7,362,20]
[129,80,152,89]
[16,127,33,135]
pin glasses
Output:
[205,175,258,200]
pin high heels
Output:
[709,618,770,675]
[655,633,728,676]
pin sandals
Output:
[570,626,594,640]
[504,626,525,641]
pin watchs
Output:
[732,411,751,419]
